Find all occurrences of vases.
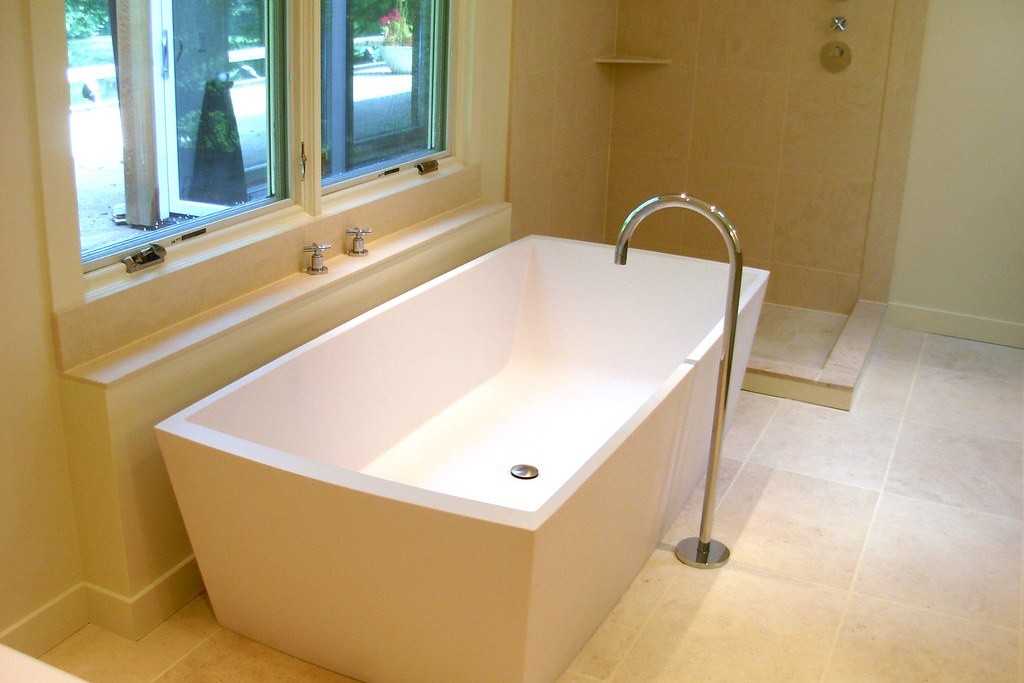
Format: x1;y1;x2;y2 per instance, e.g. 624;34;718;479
379;45;413;74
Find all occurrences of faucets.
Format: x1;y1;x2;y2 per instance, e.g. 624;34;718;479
613;193;742;284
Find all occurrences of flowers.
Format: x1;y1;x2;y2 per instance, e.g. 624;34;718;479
379;10;413;46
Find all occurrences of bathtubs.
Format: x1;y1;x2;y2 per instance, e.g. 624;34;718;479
152;231;772;683
